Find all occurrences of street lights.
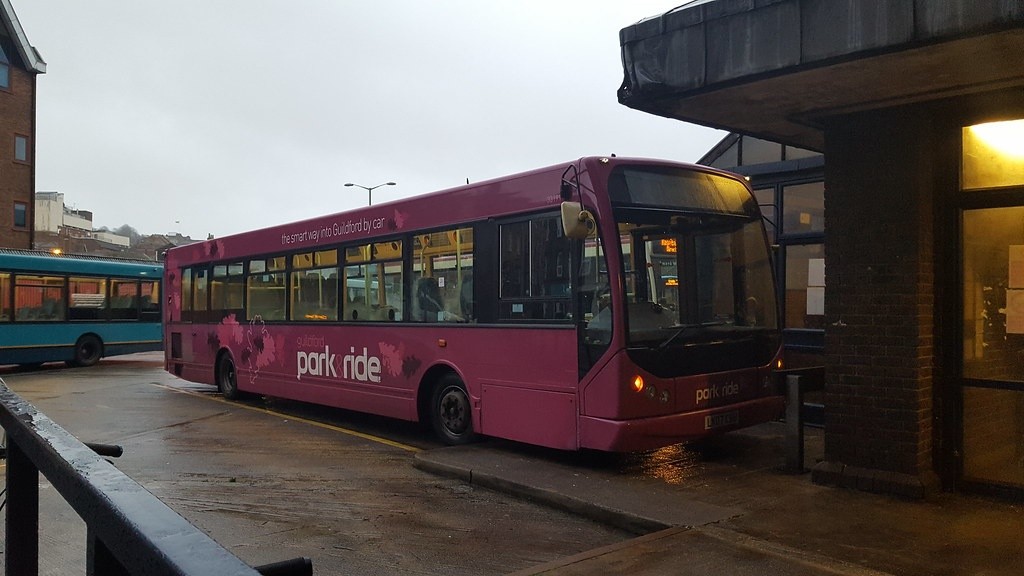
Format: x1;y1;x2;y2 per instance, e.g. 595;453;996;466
344;182;396;205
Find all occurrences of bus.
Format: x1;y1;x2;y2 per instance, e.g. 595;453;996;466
378;230;680;324
0;253;164;369
164;155;786;454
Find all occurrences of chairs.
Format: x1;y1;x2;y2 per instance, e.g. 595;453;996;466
191;274;353;323
17;293;152;321
416;278;446;323
